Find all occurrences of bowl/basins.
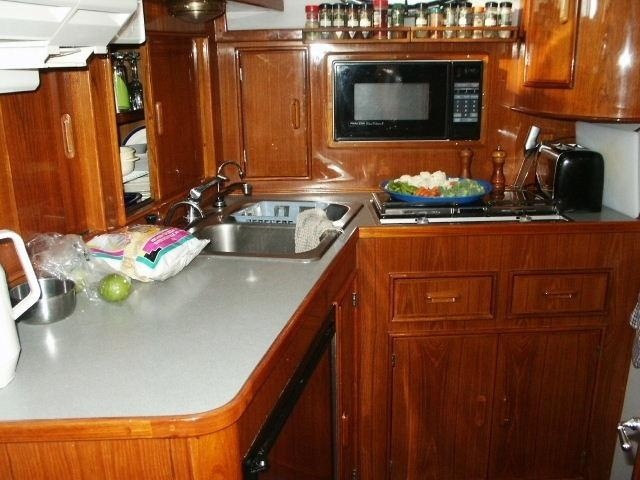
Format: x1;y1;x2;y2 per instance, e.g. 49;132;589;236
8;277;76;325
117;147;140;177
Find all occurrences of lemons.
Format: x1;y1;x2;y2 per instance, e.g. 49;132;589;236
100;274;133;303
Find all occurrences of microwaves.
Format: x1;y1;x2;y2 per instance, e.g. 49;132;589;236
332;59;484;143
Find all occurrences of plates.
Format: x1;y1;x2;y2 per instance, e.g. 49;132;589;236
381;178;493;205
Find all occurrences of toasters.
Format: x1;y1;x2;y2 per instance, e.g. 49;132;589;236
536;143;605;213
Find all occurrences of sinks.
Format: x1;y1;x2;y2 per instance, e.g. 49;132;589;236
229;197;349;226
193;224;326;257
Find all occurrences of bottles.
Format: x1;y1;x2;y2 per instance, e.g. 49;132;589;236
303;0;513;42
110;51;143;110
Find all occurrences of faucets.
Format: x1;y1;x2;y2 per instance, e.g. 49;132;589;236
214;158;244;214
143;199;204;228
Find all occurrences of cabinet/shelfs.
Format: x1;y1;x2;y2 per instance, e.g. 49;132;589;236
0;228;358;480
359;221;639;480
498;0;640;124
1;30;206;242
218;42;313;196
